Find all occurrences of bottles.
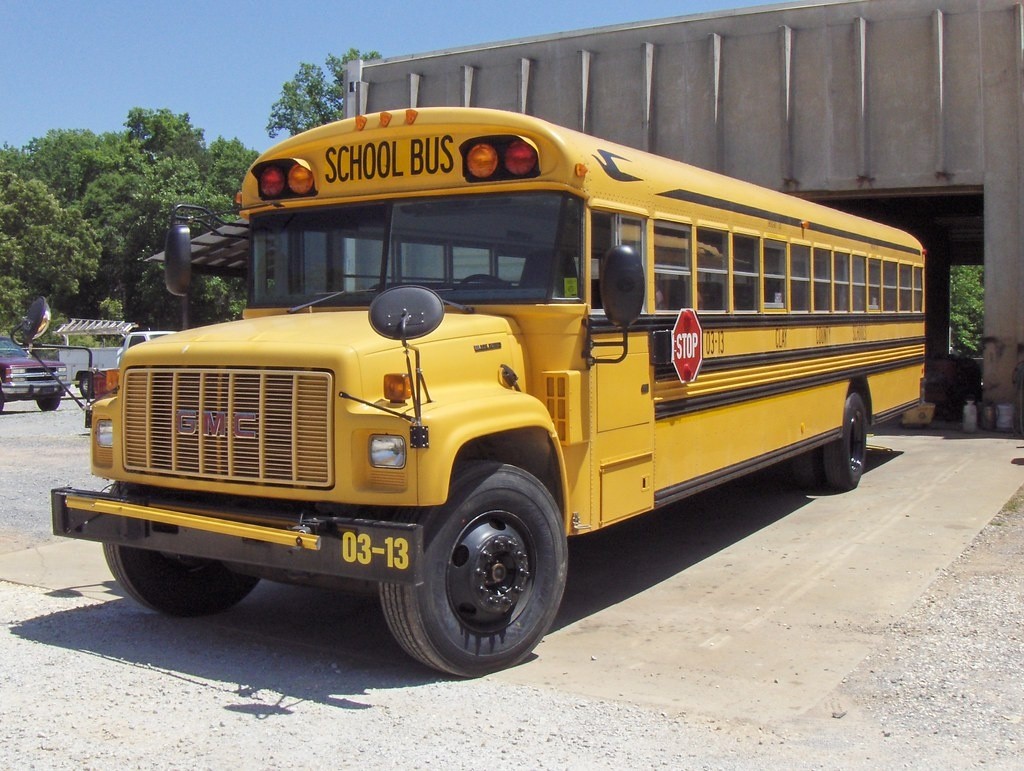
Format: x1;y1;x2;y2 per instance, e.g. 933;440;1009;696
961;395;977;433
982;399;999;431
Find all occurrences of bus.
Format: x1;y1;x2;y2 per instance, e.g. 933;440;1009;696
51;101;930;675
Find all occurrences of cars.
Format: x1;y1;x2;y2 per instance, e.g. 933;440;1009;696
124;330;178;355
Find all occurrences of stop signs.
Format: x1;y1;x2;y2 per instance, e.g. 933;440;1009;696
671;307;702;384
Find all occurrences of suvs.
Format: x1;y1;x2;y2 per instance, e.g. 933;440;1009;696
0;337;73;414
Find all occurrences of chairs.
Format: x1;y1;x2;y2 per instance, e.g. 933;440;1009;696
518;249;785;309
